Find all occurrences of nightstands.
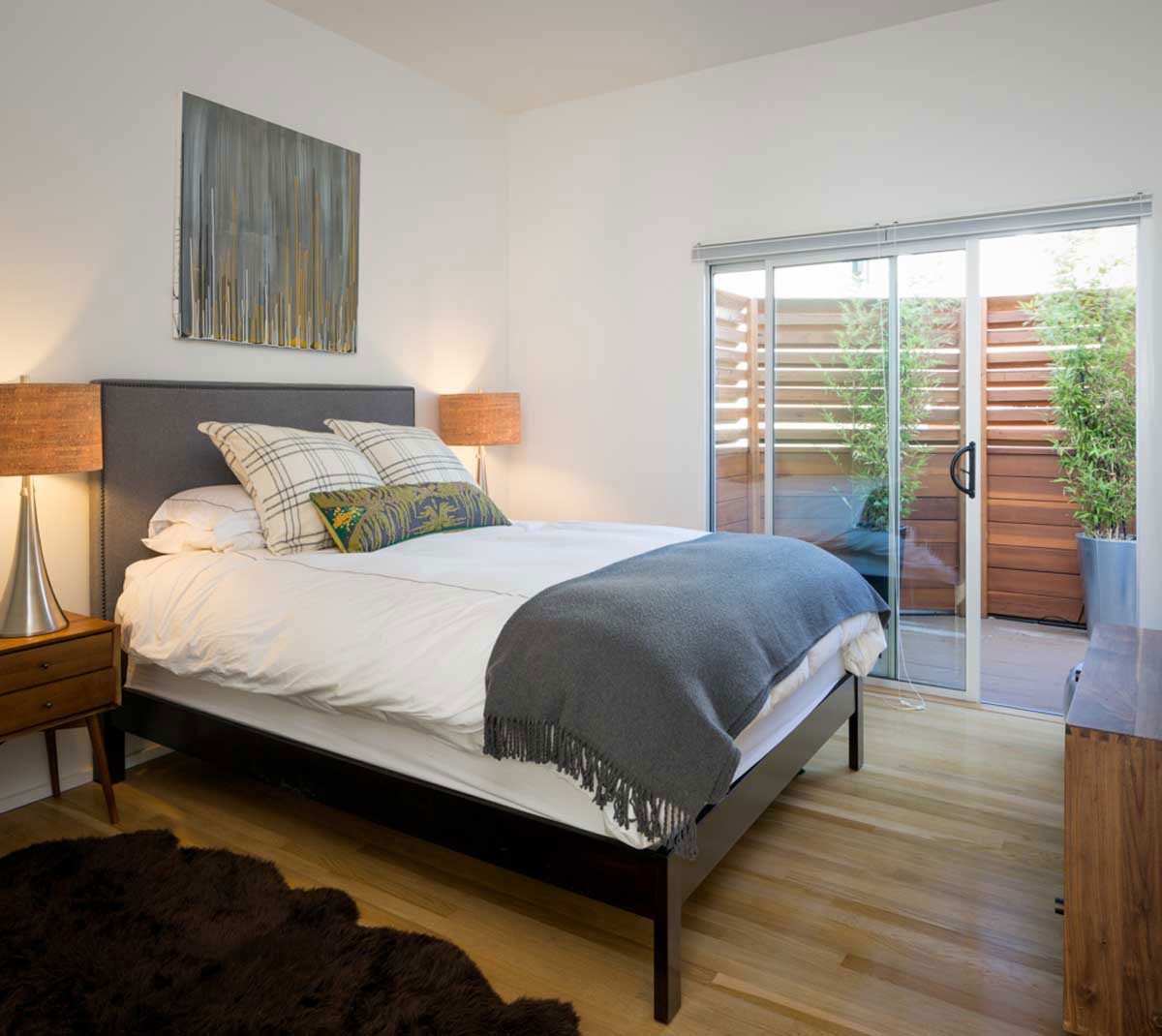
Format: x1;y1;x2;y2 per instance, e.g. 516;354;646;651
0;608;122;825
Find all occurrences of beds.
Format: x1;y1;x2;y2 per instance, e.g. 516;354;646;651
90;381;891;1025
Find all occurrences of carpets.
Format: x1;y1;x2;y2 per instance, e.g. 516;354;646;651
0;829;581;1035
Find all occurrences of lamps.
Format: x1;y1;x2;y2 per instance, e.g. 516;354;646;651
0;374;103;637
439;389;523;498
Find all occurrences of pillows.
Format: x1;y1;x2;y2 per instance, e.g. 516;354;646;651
309;482;513;553
142;485;267;555
198;420;382;554
323;417;482;492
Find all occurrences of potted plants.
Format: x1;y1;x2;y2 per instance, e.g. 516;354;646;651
1012;230;1138;642
809;273;961;630
